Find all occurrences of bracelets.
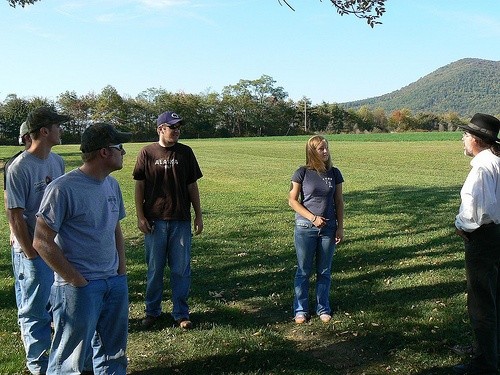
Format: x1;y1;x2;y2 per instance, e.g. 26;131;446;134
312;217;316;222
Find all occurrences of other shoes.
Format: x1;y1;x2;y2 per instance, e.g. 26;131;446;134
292;315;308;323
452;364;472;374
320;314;332;322
81;371;94;375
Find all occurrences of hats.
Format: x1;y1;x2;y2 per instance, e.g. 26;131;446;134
157;111;183;126
453;112;500;142
26;106;75;132
80;123;132;153
20;121;30;138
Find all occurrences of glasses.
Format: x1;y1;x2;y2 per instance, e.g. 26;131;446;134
107;143;122;151
166;123;181;129
463;132;471;138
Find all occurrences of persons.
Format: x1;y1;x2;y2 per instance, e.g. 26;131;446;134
33;123;132;375
4;109;73;375
289;136;345;323
132;112;203;327
455;114;500;375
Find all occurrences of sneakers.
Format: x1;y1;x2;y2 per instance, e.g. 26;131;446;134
177;317;192;328
142;315;158;325
